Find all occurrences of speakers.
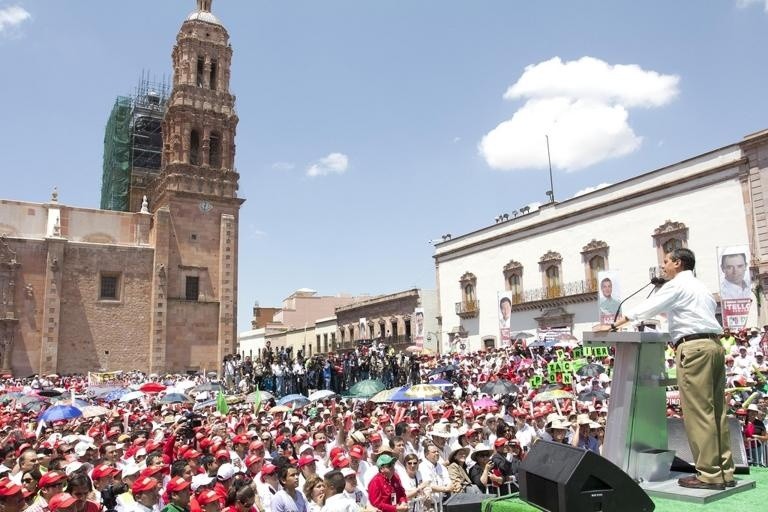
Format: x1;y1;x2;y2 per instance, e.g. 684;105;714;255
520;439;655;510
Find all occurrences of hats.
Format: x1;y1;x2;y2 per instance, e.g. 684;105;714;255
0;376;420;512
725;326;768;417
666;354;674;362
417;344;602;464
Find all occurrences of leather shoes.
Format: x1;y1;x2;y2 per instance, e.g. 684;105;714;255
677;474;737;491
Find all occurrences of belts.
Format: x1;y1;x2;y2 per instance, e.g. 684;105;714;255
674;332;720;350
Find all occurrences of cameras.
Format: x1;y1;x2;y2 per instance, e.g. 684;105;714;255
508;442;517;446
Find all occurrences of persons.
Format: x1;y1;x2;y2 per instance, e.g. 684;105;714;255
1;324;767;511
497;296;512;344
721;253;758;332
591;248;737;491
415;312;425;349
599;277;623;324
360;323;366;339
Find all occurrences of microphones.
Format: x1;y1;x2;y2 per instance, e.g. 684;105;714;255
647;278;666;299
613;277;659;330
